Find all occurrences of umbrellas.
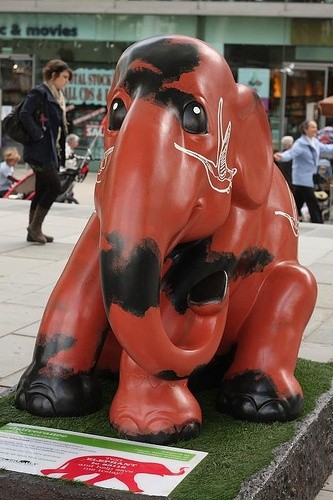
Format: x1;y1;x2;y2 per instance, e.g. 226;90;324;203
318;96;333;116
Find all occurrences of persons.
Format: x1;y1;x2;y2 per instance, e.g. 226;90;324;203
21;59;73;243
59;134;79;196
0;147;21;198
272;120;333;225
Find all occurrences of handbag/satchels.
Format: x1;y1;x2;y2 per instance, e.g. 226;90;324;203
313;174;321;184
1;98;41;146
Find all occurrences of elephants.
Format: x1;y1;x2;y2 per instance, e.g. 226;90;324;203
12;35;318;444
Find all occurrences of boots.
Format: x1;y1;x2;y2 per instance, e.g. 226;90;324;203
27;207;54;242
27;203;49;244
